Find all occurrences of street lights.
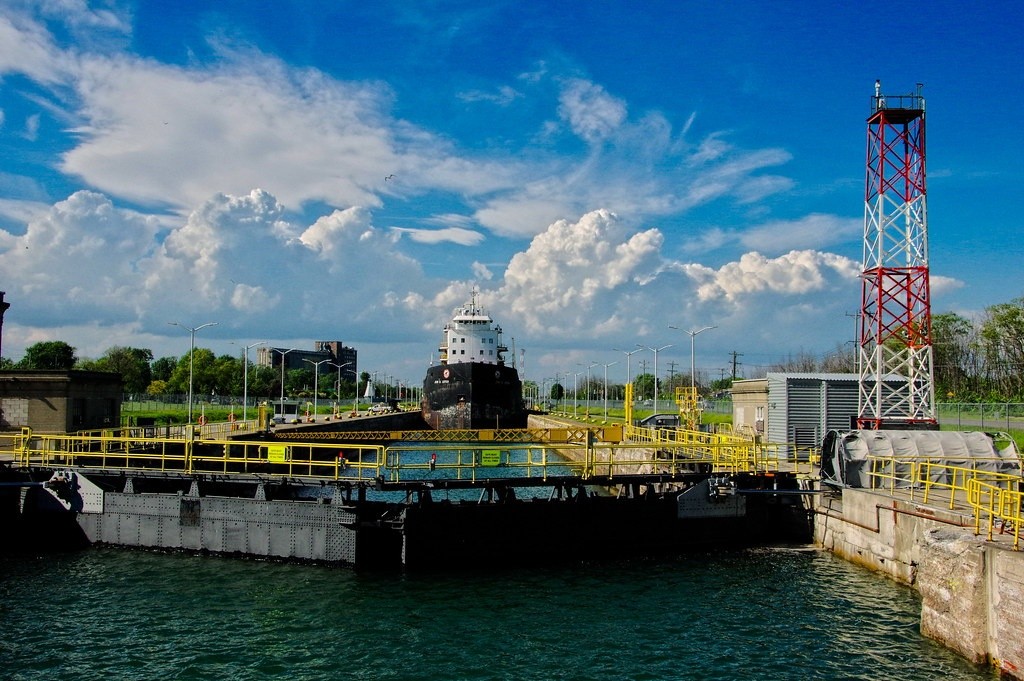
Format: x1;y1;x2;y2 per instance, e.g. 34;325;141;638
347;370;367;413
301;359;332;420
168;323;219;424
269;348;301;417
327;362;352;403
636;344;673;414
576;363;601;411
527;372;583;417
373;371;419;411
592;361;620;421
612;348;644;384
228;342;268;423
669;326;719;401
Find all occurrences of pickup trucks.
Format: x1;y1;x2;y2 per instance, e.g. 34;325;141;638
368;405;392;413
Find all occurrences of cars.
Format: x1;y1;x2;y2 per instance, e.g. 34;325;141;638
626;413;680;441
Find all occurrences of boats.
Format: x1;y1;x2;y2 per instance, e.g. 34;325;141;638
420;287;530;430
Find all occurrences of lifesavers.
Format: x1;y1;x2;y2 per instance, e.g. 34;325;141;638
199;416;208;424
334;409;337;413
305;410;312;417
228;413;238;422
